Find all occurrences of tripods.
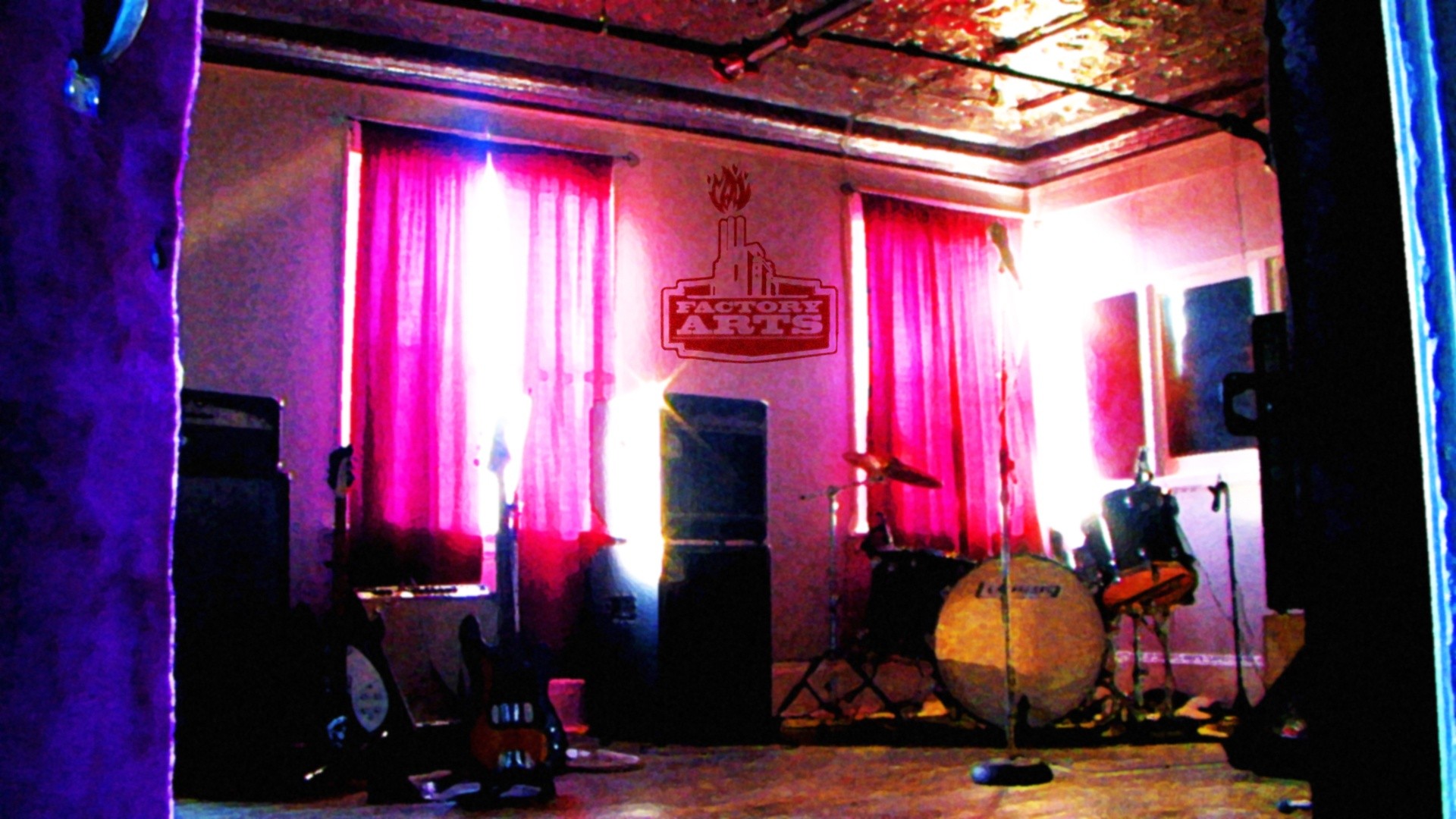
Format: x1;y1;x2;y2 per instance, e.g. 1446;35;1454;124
774;484;906;724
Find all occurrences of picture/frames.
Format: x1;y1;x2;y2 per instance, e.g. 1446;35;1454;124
1049;246;1285;486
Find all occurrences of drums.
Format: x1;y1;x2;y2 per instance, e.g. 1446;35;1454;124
838;482;1199;735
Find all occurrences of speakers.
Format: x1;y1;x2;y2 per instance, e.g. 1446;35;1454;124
179;384;281;473
657;545;772;744
660;393;769;543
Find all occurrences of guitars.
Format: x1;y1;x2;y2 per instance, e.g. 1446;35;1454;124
291;443;438;790
457;499;568;802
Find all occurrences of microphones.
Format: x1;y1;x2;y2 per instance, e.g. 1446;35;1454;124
1131;446;1147;484
1205;478;1227;512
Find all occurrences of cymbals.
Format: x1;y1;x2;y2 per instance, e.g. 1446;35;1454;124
842;450;939;488
